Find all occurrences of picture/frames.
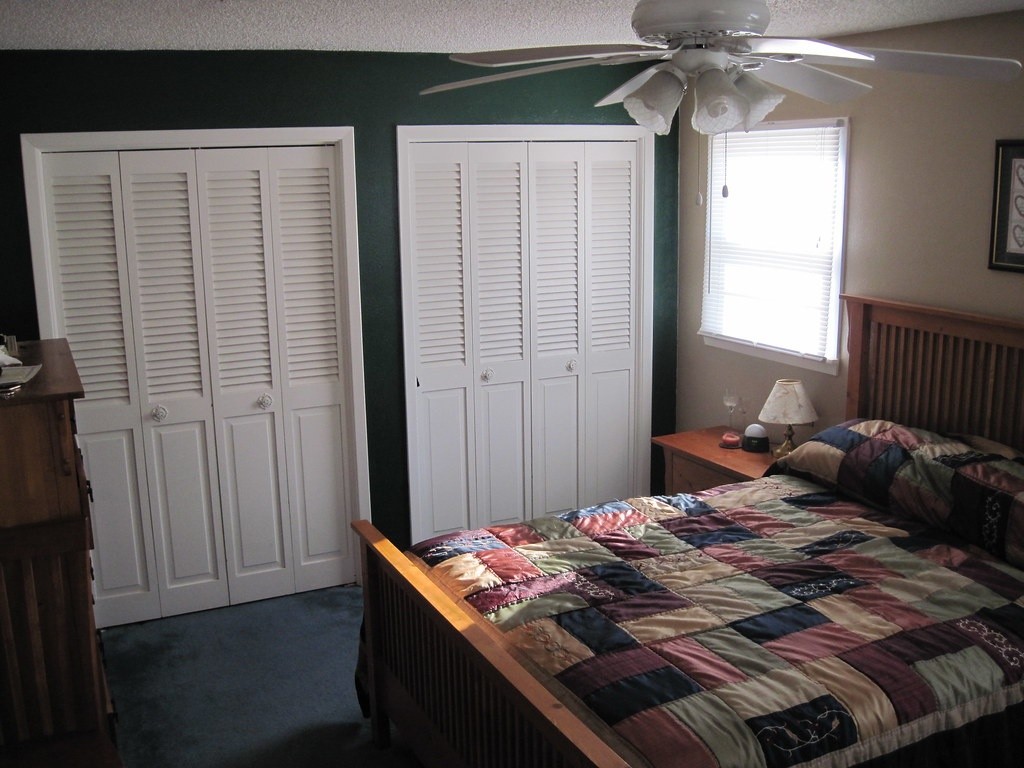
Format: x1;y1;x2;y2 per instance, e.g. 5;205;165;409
989;139;1024;270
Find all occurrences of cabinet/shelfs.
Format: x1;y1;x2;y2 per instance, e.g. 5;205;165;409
1;339;124;768
20;126;372;629
395;124;655;545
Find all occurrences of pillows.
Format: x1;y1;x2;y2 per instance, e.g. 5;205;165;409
763;417;1024;570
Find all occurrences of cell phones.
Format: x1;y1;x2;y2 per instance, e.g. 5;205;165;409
0;385;21;393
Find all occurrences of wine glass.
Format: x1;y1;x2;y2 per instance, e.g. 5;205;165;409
723;384;739;435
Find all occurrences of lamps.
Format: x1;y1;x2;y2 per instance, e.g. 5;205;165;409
757;378;819;458
623;48;786;206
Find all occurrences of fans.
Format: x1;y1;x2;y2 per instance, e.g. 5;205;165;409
419;0;1022;106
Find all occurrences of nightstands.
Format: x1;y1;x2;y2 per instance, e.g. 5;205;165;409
651;425;782;496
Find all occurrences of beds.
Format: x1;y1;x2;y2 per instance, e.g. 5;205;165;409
353;295;1024;768
